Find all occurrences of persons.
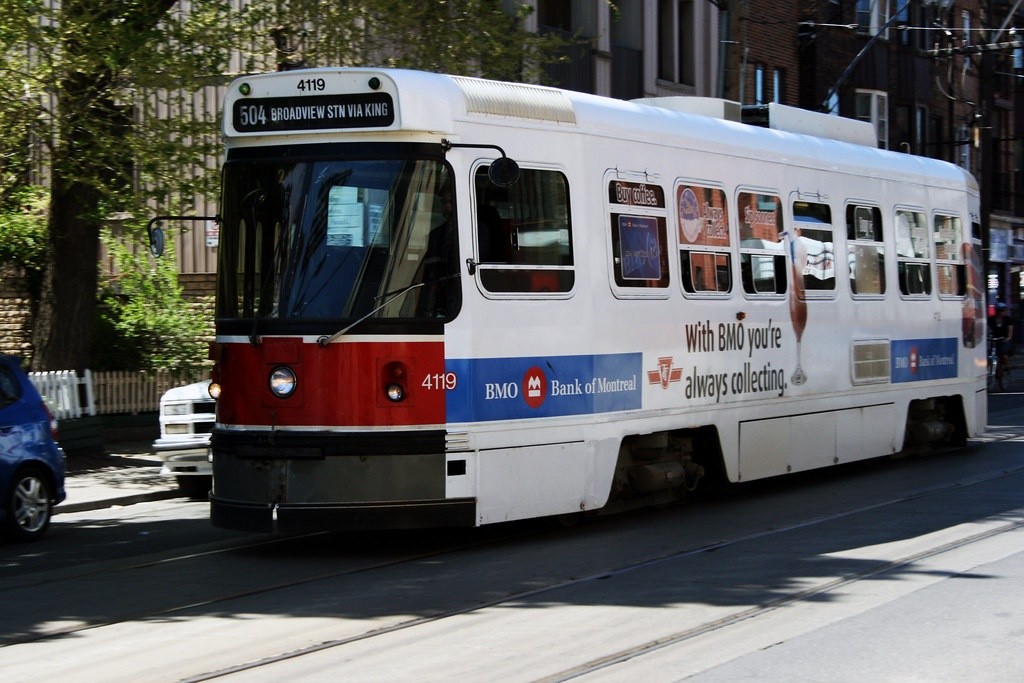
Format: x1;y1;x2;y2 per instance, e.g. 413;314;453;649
425;172;513;321
987;302;1014;370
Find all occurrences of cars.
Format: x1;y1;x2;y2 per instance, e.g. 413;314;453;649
152;380;212;503
0;352;66;547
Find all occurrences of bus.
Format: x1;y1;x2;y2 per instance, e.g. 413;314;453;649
147;67;988;537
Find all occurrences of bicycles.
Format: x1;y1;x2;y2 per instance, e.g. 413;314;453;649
985;335;1012;394
